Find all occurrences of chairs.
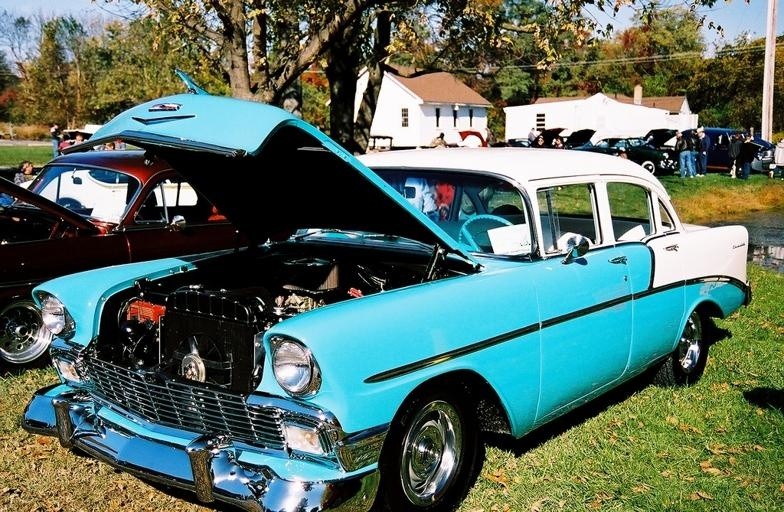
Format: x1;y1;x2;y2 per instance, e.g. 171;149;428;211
490;204;524;227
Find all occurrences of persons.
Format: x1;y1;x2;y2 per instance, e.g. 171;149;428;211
527;128;783;180
431;182;456;222
13;160;37;187
49;120;125;158
430;132;449;148
403;177;441;224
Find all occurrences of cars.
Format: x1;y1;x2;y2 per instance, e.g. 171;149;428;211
15;161;197;225
0;149;248;368
59;123;107;152
22;67;751;511
